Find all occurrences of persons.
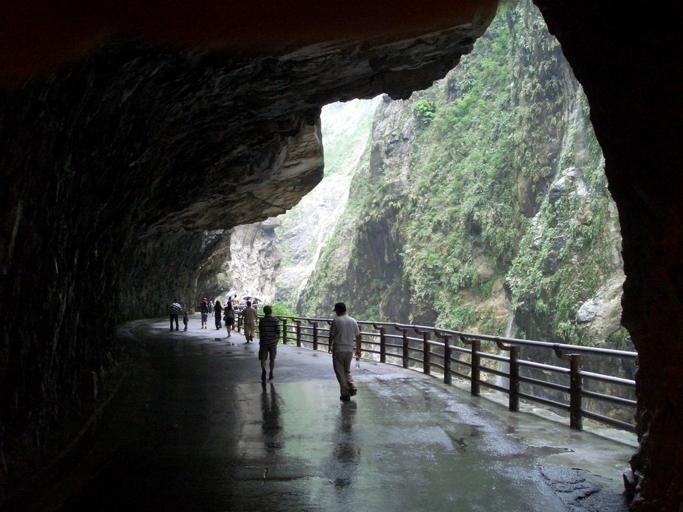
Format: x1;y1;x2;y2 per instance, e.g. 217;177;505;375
328;303;362;402
168;294;258;343
259;306;280;381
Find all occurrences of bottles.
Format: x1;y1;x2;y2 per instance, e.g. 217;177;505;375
356;354;359;369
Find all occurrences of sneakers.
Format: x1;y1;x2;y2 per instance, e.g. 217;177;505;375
351;388;357;396
247;335;253;343
340;396;350;401
262;370;273;380
